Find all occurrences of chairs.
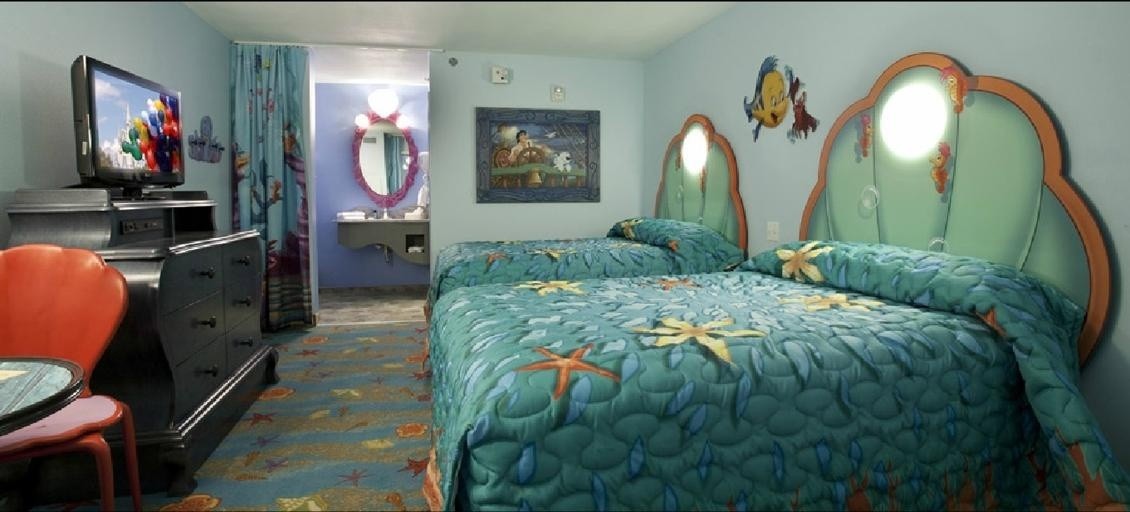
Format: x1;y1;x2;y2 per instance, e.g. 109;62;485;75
0;243;143;512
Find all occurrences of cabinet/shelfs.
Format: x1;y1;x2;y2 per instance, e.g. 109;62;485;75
5;188;281;496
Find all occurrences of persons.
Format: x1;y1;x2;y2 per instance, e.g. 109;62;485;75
508;129;536;161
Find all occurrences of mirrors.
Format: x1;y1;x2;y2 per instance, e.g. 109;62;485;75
351;111;419;208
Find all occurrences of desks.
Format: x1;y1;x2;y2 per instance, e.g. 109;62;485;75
0;353;86;436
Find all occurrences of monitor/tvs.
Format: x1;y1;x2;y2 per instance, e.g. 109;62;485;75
69;55;185;201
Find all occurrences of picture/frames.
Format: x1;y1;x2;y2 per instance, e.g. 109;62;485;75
474;105;601;204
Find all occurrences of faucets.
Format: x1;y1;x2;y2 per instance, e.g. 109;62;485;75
383;207;389;218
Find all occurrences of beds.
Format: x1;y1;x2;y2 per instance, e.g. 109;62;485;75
423;52;1111;511
435;112;748;297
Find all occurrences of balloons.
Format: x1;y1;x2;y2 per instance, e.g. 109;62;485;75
120;93;182;174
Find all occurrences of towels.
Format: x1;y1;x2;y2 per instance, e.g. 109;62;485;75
336;210;365;221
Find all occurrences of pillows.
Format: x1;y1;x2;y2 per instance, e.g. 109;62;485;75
607;217;746;274
733;238;1130;511
